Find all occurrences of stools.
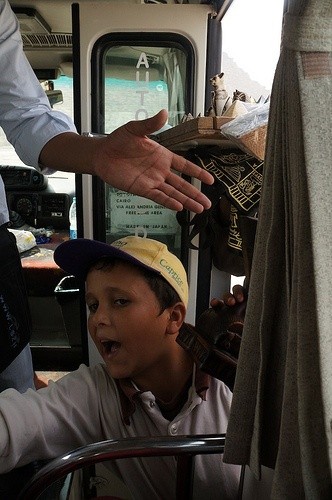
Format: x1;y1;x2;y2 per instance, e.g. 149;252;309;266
19;228;78;349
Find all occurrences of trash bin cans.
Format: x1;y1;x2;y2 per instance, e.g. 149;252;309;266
54;275;82;347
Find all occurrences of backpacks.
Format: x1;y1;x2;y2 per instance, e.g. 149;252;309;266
176;145;264;276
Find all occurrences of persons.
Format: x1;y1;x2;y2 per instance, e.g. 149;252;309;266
0;236;243;500
0;0;214;499
210;284;243;308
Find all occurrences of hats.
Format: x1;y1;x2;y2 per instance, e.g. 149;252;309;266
53;236;188;312
